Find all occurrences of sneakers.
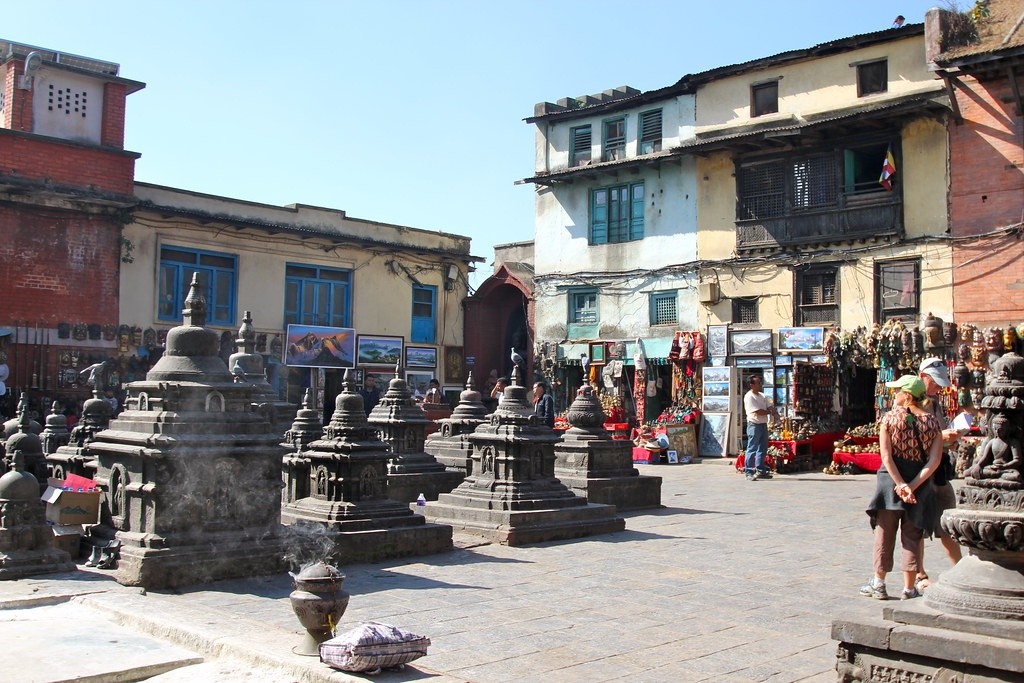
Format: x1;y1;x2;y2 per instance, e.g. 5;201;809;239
899;590;921;601
859;578;888;599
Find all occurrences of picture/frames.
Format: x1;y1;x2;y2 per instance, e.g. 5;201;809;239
405;370;433;396
443;345;464;383
591;342;608;366
356;335;404;369
665;324;824;462
363;372;397;397
280;324;356;370
405;345;437;367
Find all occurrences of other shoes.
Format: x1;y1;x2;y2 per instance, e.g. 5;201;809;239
920;575;929;589
755;472;774;479
746;473;757;481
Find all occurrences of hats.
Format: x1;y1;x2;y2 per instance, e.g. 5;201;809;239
885;375;926;402
920;357;952;387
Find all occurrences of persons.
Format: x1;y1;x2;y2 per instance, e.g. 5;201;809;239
64;406;78;429
360;373;383;417
911;356;963;596
422;377;449;404
491;377;511;405
862;374;944;603
742;375;780;481
532;382;555;428
102;386;118;412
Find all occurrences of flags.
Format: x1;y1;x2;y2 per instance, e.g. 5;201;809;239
879;142;896;191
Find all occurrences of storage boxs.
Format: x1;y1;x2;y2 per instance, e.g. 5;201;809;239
40;477;104;526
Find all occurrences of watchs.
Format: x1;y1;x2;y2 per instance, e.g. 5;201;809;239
768;409;773;413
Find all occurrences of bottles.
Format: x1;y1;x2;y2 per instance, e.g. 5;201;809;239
416;493;426;515
56;486;94;492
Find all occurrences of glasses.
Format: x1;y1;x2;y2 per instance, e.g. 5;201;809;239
923;361;947;372
